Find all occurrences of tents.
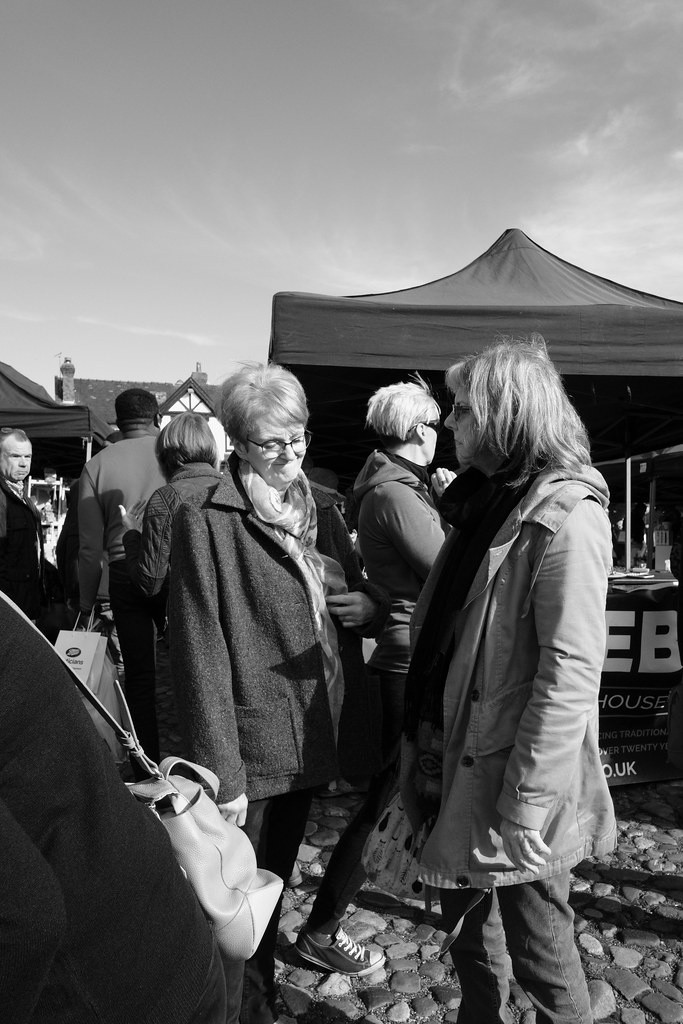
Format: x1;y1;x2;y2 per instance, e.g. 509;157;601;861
0;361;118;462
266;228;683;573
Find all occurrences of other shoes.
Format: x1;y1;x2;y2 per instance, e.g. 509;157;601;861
288;860;303;887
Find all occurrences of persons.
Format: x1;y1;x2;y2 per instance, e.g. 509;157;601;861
0;342;617;1024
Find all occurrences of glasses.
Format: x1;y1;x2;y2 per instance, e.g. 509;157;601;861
452;404;473;421
409;421;441;435
247;429;312;458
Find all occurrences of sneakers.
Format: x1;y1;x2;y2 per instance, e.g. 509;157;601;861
294;922;387;976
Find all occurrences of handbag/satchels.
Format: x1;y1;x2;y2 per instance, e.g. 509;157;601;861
123;755;284;961
361;792;441;902
54;604;108;694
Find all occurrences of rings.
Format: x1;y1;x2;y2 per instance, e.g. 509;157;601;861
522;848;533;855
135;516;138;519
